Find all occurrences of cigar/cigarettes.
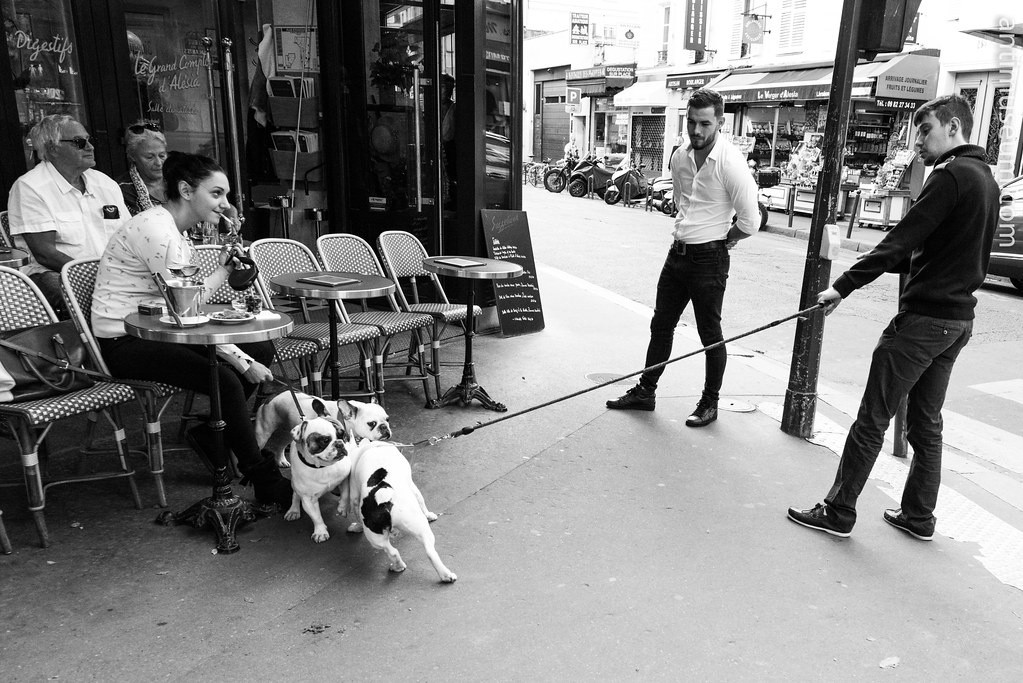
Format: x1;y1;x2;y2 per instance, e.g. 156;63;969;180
219;212;236;226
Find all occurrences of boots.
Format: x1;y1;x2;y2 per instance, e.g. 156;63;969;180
239;449;293;514
184;422;234;482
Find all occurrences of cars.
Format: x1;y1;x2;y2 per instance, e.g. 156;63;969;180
987;175;1023;292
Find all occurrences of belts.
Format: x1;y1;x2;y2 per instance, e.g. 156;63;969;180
674;240;726;255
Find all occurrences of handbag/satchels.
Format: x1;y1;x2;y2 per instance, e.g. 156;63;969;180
0;319;95;404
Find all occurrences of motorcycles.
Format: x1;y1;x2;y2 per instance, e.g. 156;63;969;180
544;138;782;230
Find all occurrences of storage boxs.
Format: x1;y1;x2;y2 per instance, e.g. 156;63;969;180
137;303;166;316
757;166;781;186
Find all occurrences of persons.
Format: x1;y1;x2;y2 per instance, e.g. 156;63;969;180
786;93;999;538
7;114;132;323
111;121;171;217
606;87;762;428
93;149;294;512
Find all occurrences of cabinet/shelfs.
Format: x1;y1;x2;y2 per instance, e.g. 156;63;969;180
747;131;888;164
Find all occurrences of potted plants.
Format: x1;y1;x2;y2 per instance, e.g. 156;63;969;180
365;29;423;105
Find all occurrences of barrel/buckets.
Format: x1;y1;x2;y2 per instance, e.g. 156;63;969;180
164;279;205;317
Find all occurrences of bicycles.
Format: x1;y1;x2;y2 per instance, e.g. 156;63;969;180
522;156;566;188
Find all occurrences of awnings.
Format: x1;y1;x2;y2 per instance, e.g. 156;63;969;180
614;80;673;107
699;49;940;101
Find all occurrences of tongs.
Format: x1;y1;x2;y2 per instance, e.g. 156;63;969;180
151;272;183;326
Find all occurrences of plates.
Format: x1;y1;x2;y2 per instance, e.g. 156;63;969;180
206;312;254;324
159;316;210;328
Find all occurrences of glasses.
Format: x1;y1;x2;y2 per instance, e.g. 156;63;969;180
128;123;163;134
60;138;97;149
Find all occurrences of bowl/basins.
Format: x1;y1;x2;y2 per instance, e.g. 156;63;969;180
231;299;248;311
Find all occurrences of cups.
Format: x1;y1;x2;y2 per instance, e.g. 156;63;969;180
244;294;262;315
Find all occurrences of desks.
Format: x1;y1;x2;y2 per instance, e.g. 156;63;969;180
0;247;31;271
122;304;293;553
423;254;524;412
269;271;395;402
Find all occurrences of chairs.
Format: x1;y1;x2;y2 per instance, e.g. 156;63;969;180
376;228;481;393
0;201;138;254
315;234;437;410
249;238;385;422
0;266;168;549
60;258;189;508
192;241;321;422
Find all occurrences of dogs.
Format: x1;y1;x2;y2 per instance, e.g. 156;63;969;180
284;400;357;542
353;439;457;583
255;390;393;468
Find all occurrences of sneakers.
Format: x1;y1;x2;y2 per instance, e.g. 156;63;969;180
606;384;655;411
787;503;851;537
686;395;718;427
883;508;933;541
